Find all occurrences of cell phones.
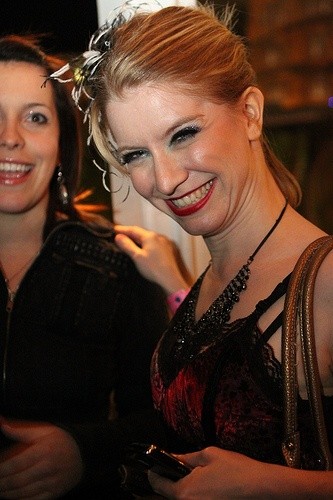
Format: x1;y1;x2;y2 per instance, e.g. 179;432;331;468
122;442;193;482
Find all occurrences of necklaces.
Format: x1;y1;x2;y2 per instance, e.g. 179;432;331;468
1;246;42;284
172;197;288;360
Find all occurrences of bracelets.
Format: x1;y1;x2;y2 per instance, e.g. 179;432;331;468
167;288;192;312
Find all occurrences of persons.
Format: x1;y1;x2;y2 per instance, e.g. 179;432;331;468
84;5;333;500
0;34;170;500
